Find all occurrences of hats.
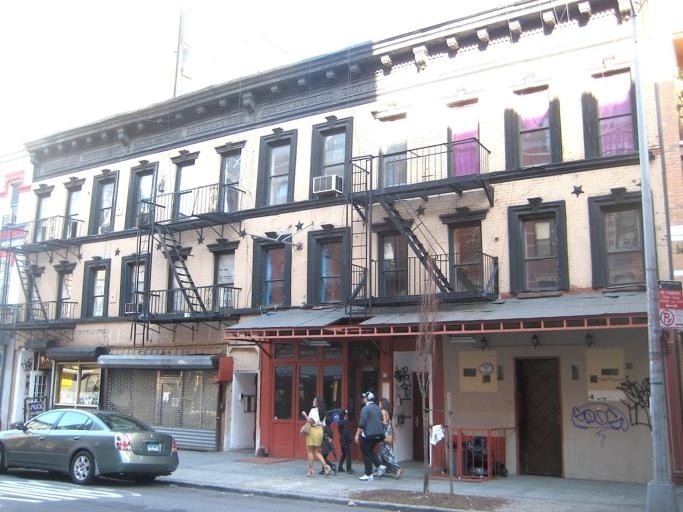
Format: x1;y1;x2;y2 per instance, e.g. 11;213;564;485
362;392;374;400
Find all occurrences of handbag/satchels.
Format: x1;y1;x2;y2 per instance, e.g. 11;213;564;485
383;434;392;444
300;423;311;435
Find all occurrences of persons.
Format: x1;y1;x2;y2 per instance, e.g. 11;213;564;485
335;407;355;475
299;395;332;479
372;396;404;480
353;390;386;482
319;415;339;475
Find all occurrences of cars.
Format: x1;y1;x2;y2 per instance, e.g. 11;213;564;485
0;407;181;485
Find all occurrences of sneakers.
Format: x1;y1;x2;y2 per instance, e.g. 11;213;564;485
395;469;402;479
358;474;372;481
376;466;386;478
306;464;354;477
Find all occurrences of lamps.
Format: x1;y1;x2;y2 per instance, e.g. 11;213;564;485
451;334;476;342
481;337;487;352
532;334;541;349
586;333;593;347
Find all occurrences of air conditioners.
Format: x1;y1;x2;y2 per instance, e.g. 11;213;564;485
313;176;343;194
125;302;143;314
